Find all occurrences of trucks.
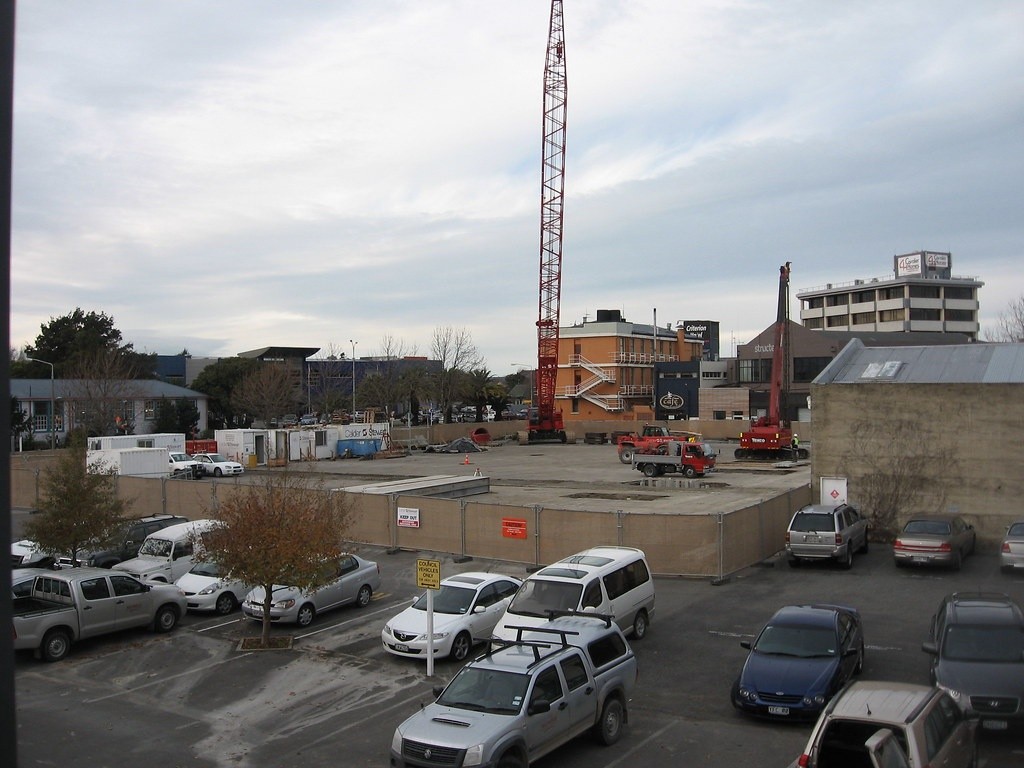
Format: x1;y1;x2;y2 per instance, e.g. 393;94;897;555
630;441;721;478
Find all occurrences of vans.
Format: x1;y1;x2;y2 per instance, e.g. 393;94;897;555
490;545;657;651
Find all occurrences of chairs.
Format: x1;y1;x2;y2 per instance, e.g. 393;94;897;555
484;672;516;703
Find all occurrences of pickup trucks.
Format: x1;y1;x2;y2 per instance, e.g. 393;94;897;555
11;565;188;662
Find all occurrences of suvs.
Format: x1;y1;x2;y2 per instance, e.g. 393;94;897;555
794;679;977;768
388;609;639;768
11;513;234;585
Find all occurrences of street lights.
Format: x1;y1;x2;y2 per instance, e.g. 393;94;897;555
510;363;533;409
349;339;358;422
24;357;55;449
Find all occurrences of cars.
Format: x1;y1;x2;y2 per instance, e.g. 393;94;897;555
920;590;1024;733
381;570;525;661
264;405;529;429
171;549;256;613
736;603;862;722
997;521;1024;573
169;452;244;476
785;503;870;570
894;515;978;572
242;552;382;628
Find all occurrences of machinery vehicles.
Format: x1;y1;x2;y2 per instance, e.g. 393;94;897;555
735;261;812;461
515;0;576;447
616;424;678;465
